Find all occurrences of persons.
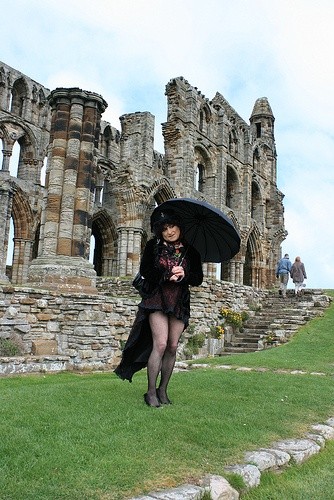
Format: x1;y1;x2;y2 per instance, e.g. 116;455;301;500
113;217;204;408
290;257;307;296
276;254;292;298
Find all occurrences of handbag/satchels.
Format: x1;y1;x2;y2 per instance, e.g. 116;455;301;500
132;270;159;299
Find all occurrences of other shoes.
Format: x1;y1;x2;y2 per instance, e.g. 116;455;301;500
278;290;282;295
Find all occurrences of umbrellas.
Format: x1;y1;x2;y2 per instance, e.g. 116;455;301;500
150;198;241;282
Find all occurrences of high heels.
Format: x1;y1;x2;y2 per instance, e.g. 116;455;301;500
155;387;172;405
144;393;162;408
